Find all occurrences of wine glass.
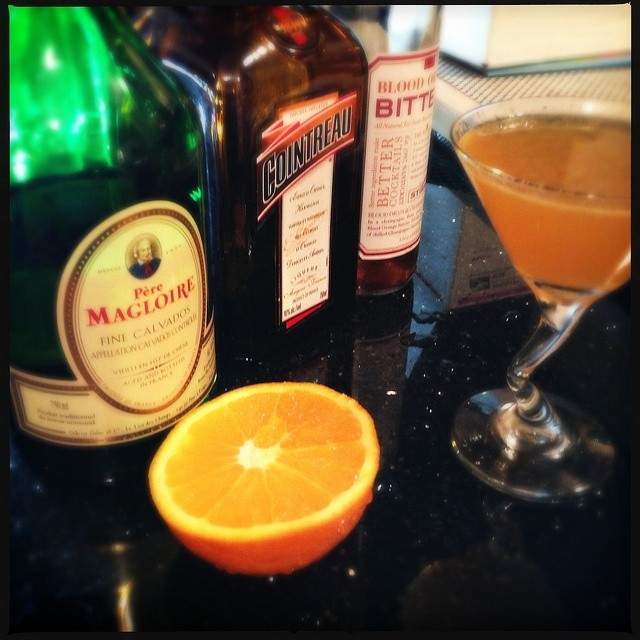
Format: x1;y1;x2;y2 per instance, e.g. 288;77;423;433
449;96;631;503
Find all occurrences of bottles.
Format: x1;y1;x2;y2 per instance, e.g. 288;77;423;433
7;3;223;498
318;2;445;298
131;5;368;346
